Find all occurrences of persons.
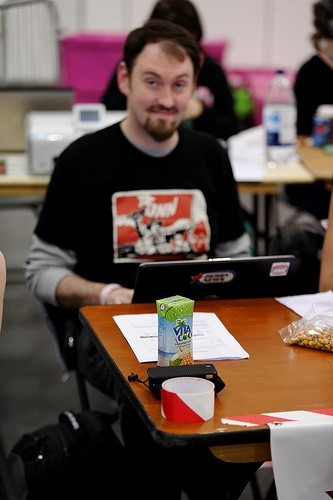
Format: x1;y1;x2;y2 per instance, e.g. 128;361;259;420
24;21;264;500
100;1;238;140
283;1;332;292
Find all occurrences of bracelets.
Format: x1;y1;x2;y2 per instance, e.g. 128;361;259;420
98;283;120;306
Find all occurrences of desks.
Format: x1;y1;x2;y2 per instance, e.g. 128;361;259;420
0;136;333;447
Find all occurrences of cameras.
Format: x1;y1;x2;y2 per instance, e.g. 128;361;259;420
146;363;225;399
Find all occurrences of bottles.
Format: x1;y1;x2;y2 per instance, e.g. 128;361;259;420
262;70;296;170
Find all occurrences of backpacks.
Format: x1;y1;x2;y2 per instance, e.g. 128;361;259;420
0;408;132;500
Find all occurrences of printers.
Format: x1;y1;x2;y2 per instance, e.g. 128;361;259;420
25;104;130;176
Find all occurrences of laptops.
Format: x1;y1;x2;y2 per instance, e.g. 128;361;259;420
132;255;296;304
